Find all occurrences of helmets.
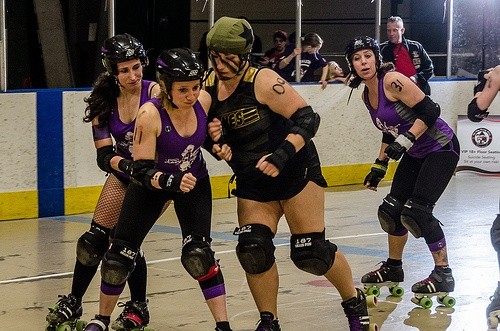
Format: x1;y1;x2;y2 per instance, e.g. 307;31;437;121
206;17;254;55
100;34;149;76
157;48;204;83
343;36;381;72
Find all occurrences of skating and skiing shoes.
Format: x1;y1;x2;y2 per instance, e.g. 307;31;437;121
111;299;151;331
45;293;86;331
82;314;111;331
486;296;500;329
411;265;456;308
254;311;282;331
341;287;379;331
360;260;405;298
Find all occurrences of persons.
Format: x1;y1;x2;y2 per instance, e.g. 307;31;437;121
84;46;237;331
258;32;349;91
44;35;164;331
203;15;370;331
468;60;500;124
380;14;436;99
345;35;460;294
